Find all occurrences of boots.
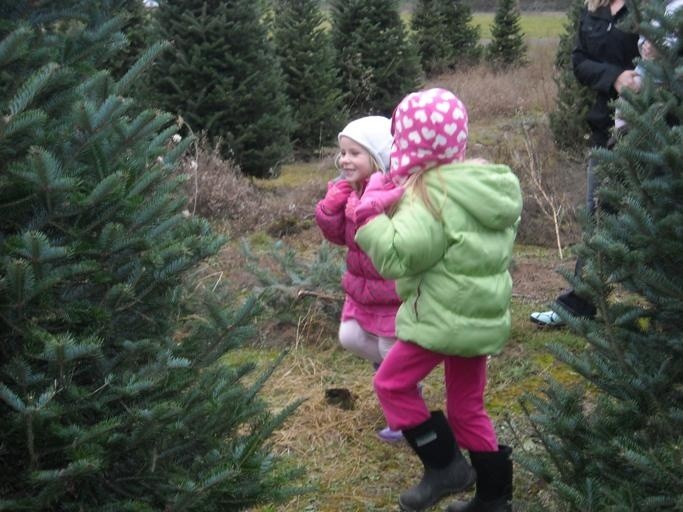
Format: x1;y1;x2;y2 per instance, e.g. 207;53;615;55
399;409;477;512
446;445;512;512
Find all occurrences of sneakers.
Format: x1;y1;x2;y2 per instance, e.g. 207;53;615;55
379;426;402;440
530;311;561;327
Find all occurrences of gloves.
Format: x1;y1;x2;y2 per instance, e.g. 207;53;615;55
322;172;404;223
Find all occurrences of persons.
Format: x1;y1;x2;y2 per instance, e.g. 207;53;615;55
605;2;683;141
312;116;429;442
529;1;663;329
352;86;523;511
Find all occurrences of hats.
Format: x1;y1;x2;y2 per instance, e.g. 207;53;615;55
390;89;468;169
337;115;389;176
